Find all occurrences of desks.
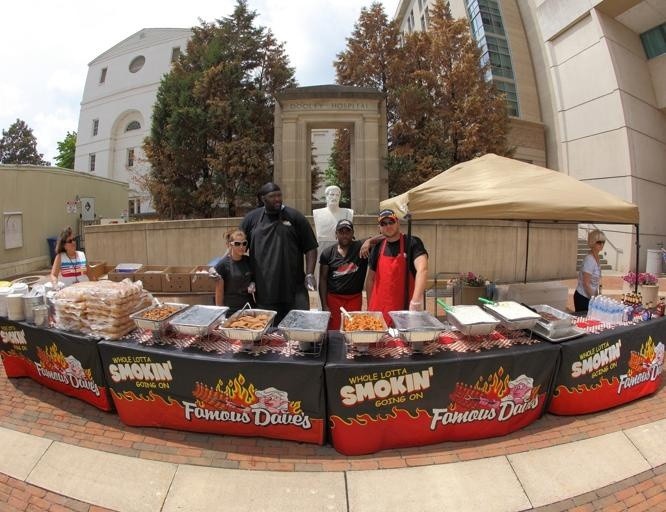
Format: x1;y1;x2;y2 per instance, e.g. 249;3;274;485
150;291;216;306
544;307;666;417
322;329;563;458
96;328;330;447
0;316;137;413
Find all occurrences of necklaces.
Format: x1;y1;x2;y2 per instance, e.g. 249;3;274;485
66;252;77;281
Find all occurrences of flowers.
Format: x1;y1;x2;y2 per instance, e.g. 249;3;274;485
621;269;659;286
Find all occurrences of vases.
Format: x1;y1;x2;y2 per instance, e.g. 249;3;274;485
640;284;659;309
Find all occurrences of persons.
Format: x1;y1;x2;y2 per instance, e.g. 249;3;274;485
364;209;429;314
208;182;319;326
50;226;97;297
318;219;387;330
312;185;354;267
574;229;607;312
213;228;257;318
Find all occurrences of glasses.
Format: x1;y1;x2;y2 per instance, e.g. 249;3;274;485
595;241;605;244
379;220;396;227
229;240;248;248
65;237;76;243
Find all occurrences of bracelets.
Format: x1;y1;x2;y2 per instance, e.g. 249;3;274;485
410;300;423;306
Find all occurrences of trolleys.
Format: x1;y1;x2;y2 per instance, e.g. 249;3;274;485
423;271;463;326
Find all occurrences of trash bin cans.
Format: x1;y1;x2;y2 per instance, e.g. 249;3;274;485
46;237;57;265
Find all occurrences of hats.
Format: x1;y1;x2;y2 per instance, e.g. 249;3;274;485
336;219;354;232
377;209;398;224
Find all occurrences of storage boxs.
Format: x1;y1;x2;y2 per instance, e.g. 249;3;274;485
161;265;196;292
86;260;109;282
190;264;216;292
135;264;167;292
108;265;143;283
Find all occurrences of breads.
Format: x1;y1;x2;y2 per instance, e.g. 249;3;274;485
51;278;153;343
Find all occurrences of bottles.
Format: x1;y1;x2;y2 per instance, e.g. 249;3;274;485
587;295;628;325
621;291;665;321
